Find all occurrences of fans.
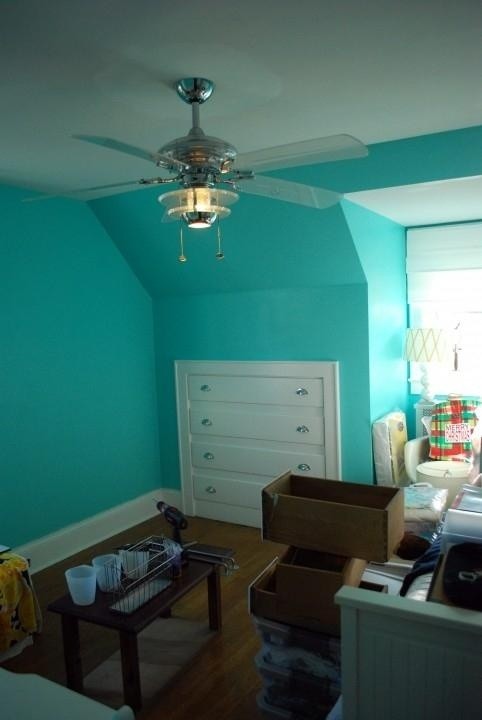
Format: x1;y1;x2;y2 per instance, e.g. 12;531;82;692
18;75;367;263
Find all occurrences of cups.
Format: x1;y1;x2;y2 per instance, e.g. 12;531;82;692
64;544;150;605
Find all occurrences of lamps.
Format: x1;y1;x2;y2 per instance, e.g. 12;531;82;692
157;186;240;232
404;328;448;404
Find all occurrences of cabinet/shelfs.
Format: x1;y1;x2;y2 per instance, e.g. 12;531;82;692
175;359;339;527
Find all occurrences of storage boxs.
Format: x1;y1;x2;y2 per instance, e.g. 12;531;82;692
247;470;405;719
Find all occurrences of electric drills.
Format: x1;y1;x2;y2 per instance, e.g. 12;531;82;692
151;496;188;567
